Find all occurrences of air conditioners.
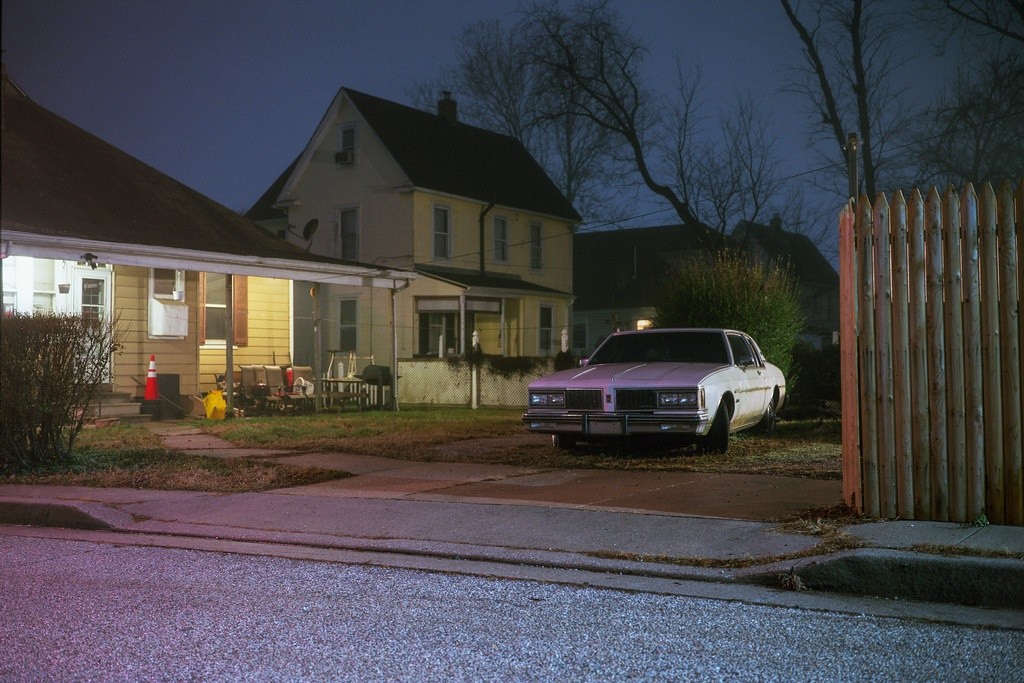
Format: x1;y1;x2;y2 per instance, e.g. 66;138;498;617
335;150;352;164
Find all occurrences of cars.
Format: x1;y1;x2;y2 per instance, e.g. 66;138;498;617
521;328;786;454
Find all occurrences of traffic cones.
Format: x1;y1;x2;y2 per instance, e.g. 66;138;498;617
138;356;162;404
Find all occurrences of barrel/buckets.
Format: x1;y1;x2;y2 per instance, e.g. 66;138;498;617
172;284;184;301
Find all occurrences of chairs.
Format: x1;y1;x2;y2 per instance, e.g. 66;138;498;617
239;364;327;416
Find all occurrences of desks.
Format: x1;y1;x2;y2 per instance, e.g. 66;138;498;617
313;378;364;412
